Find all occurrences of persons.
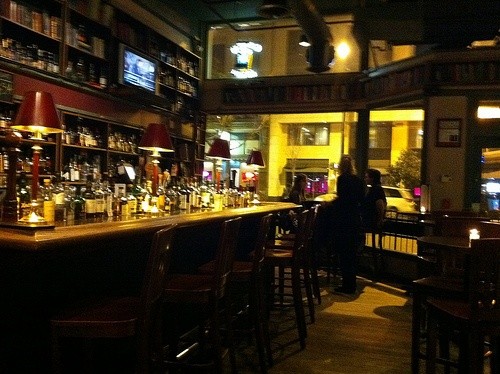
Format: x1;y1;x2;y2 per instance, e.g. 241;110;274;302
333;153;365;293
363;168;387;227
285;173;309;228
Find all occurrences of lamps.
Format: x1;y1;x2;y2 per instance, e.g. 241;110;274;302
10;89;64;224
246;150;265;204
207;139;232;193
140;120;177;214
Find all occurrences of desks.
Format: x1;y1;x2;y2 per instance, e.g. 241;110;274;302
417;235;497;274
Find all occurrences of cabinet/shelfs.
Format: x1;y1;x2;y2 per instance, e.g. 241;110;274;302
0;0;204;187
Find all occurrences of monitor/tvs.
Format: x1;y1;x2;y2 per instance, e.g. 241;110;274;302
118;43;160;97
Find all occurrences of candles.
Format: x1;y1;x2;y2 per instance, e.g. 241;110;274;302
469;229;480;249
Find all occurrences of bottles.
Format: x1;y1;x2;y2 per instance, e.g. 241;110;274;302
1;0;255;228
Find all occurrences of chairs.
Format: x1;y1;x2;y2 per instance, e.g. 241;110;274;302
410;237;500;374
49;200;386;374
